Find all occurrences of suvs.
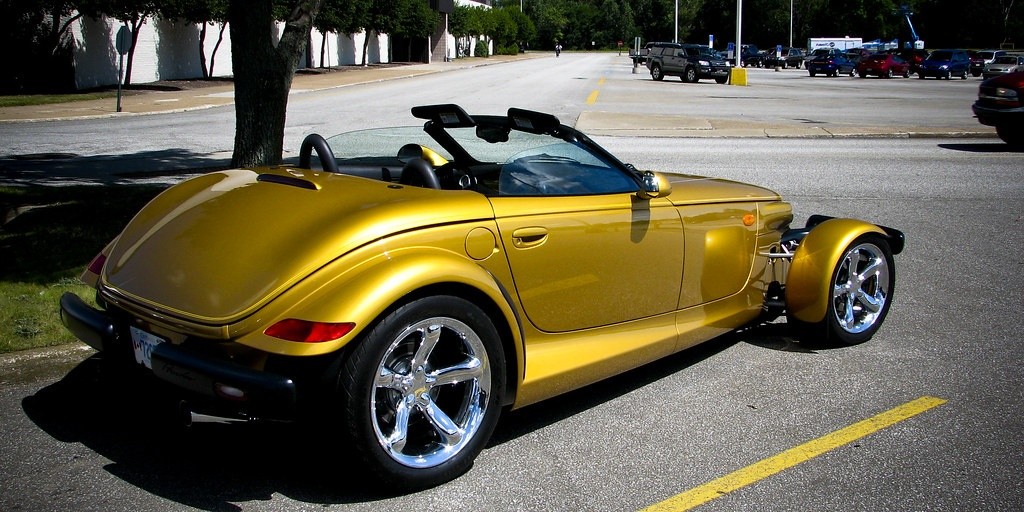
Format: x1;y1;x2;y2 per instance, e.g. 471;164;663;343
766;48;804;69
880;47;930;77
804;48;842;70
971;72;1024;153
981;49;1008;70
646;44;731;84
964;50;985;77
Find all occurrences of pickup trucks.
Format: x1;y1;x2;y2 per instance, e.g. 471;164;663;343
628;42;671;65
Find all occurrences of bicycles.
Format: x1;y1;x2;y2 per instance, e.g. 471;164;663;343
555;48;561;56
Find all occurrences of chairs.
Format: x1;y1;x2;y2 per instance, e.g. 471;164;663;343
299;133;444;190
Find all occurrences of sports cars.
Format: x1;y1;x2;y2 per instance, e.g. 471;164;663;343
57;105;903;488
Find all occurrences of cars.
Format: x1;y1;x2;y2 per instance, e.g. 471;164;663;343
519;45;525;53
919;50;971;80
982;55;1024;79
719;44;775;68
808;54;858;77
858;54;911;79
841;48;875;59
